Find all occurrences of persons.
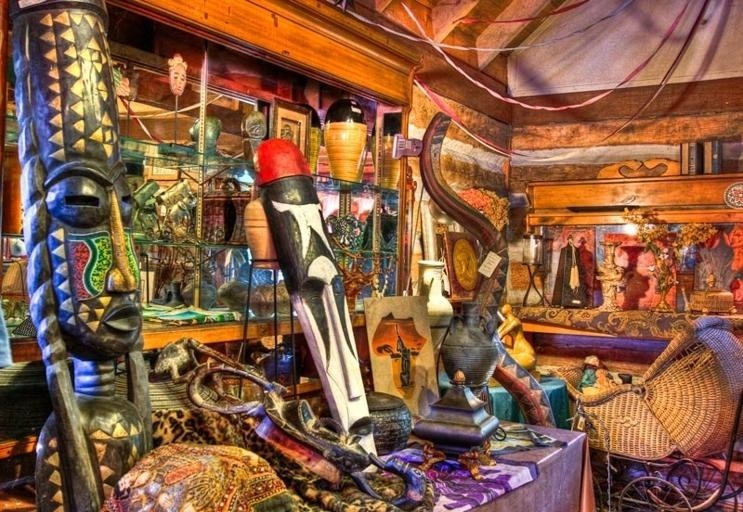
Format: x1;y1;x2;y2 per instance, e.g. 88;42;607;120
168;55;188;96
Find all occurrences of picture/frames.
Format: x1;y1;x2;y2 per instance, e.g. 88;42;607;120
270;93;312;169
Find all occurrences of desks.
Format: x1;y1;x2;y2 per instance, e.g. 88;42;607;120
311;412;588;512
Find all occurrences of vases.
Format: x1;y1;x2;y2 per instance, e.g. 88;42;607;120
322;99;368;184
243;196;281;270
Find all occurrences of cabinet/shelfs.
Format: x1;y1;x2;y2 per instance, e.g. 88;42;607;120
0;0;426;363
